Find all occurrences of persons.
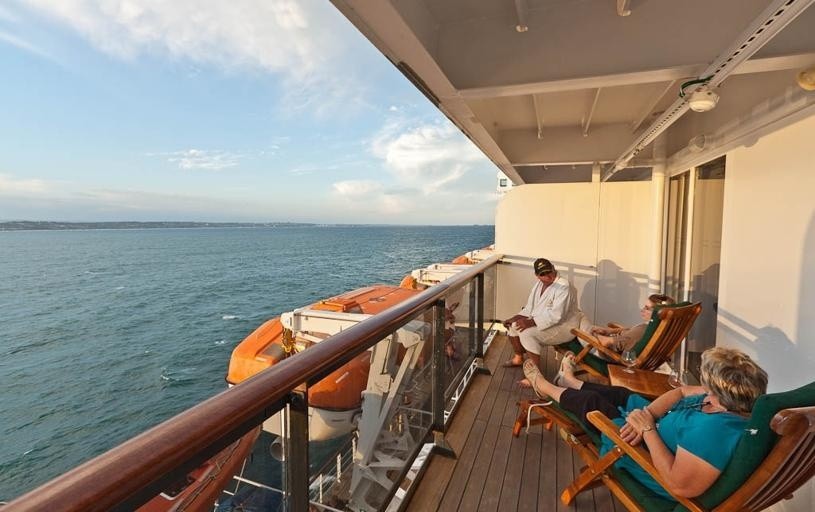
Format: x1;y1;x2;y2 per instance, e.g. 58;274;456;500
522;345;770;501
578;294;675;362
501;258;584;387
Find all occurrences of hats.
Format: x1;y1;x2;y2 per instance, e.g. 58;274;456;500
532;255;554;276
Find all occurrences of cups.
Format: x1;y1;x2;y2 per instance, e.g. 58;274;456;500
667;366;689;388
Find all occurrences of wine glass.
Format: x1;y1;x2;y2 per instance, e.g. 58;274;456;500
620;348;637;374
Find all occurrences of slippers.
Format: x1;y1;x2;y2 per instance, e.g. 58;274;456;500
502;358;523;367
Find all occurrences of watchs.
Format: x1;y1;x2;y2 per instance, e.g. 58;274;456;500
641;424;658;435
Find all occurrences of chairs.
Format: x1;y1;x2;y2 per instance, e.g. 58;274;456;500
512;395;814;511
554;302;702;388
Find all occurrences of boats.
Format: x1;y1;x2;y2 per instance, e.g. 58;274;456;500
222;277;455;443
400;240;497;331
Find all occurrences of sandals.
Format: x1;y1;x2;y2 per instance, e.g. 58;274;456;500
523;358;550;402
553;350;577;387
